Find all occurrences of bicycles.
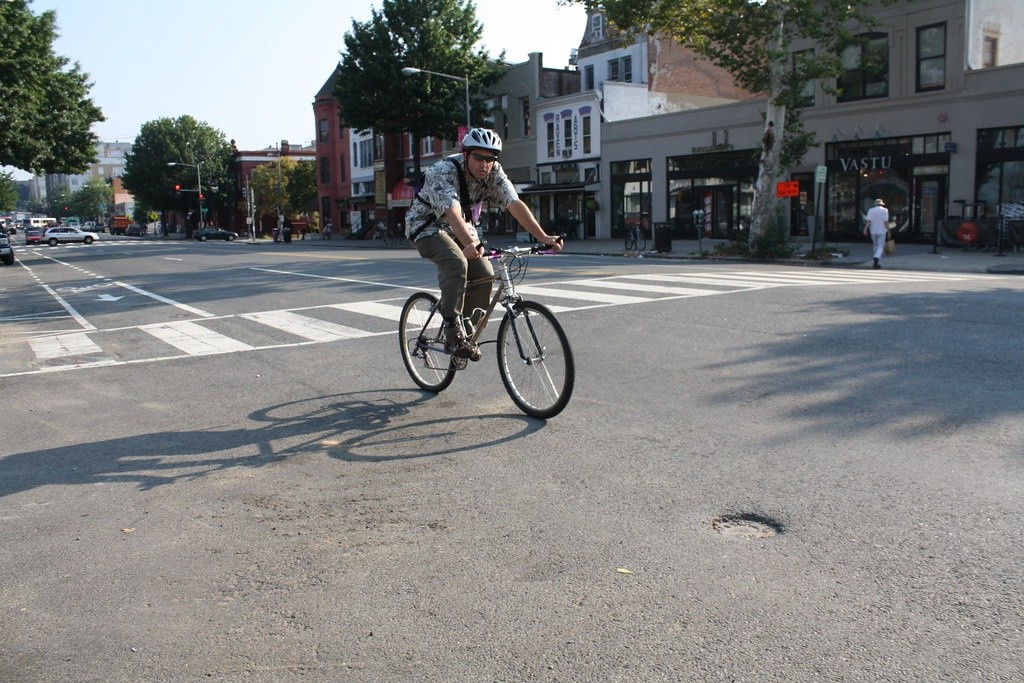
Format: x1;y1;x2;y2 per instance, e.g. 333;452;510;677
398;233;576;421
624;222;648;252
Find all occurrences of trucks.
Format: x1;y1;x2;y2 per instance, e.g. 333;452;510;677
110;216;134;235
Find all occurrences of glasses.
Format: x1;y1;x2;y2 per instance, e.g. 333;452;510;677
468;152;495;163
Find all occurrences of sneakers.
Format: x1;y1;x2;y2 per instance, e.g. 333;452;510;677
467;332;481;361
444;332;480;360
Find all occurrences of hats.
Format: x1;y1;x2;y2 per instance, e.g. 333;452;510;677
872;198;884;205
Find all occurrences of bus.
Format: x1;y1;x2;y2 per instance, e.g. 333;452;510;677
21;217;57;229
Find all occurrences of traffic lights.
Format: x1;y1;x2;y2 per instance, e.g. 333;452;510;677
252;205;257;213
174;183;182;199
65;207;68;214
199;194;206;206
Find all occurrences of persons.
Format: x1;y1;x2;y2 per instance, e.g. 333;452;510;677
405;128;564;362
387;223;405;246
863;199;891;269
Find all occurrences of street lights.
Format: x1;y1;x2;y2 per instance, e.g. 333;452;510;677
167;161;203;229
403;65;473;136
266;151;287;241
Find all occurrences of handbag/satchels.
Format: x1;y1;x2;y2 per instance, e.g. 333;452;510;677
885;240;895;255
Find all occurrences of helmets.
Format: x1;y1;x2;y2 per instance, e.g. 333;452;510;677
461;127;502;155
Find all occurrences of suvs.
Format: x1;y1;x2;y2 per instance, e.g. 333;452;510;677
40;227;100;245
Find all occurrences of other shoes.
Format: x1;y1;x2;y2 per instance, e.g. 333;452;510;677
873;257;881;269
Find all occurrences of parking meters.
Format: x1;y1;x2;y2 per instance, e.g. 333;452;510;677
692;209;706;257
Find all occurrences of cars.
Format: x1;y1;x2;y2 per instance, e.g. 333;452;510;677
125;224;145;236
26;230;42;245
0;234;15;264
196;224;239;242
0;217;22;235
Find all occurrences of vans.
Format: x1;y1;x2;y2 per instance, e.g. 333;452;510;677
60;217;106;233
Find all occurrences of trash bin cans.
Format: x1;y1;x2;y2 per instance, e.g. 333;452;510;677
272;228;279;241
284;228;291;242
654;222;672;252
